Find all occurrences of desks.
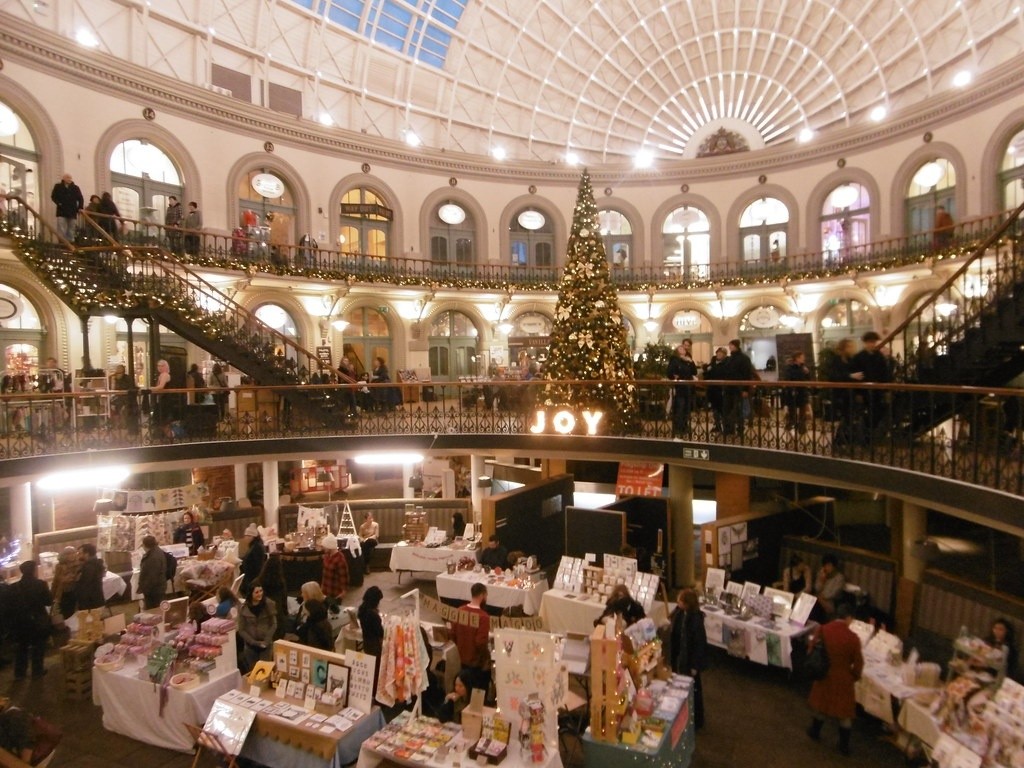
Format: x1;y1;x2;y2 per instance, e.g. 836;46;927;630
1;532;1024;768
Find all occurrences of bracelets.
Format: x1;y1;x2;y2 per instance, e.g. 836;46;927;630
176;223;179;225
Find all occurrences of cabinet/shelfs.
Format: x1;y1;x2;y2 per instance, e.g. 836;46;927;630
944;625;1009;703
71;369;110;443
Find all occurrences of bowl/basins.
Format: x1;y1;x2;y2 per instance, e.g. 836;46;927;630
169;673;200;690
94;654;122;671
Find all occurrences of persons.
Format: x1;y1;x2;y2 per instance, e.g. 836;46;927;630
782;553;845;613
966;617;1015;677
488;355;544;411
110;359;230;441
666;338;698;439
87;192;124;241
312;357;389;412
0;493;707;768
184;201;203;254
767;355;776;370
932;205;955;246
165;196;184;251
784;330;889;449
46;358;72;407
702;338;761;434
51;174;84;244
299;233;318;269
806;605;864;753
232;209;270;260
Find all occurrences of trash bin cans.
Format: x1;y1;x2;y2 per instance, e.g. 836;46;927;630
423;385;434;402
182;404;218;437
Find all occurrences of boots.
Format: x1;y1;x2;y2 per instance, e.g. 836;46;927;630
805;717;823;741
834;727;852;756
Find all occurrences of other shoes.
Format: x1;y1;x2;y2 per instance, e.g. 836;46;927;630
34;668;48;678
15;665;28;677
363;564;370;574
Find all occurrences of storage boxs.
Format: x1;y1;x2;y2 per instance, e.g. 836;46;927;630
461;704;497;740
315;701;341;715
468;715;512;766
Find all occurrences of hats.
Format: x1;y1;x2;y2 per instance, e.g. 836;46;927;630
321;533;338;550
244;523;258;537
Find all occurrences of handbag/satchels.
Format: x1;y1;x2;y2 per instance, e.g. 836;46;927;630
163;552;178;580
248;577;259;589
48;602;66;631
676;662;705;692
802;626;829;680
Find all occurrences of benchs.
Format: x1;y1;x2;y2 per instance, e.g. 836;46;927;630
772;573;868;619
